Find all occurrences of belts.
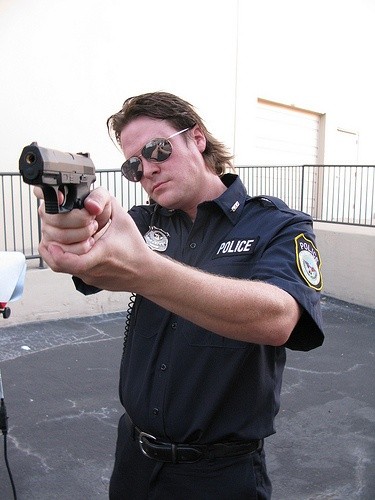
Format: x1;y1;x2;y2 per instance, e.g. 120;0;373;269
125;411;263;463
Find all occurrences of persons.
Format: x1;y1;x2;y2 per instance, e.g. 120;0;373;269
34;91;326;500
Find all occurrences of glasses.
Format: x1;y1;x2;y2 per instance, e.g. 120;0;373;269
121;127;190;182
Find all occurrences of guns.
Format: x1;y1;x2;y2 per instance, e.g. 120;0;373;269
19;141;97;214
